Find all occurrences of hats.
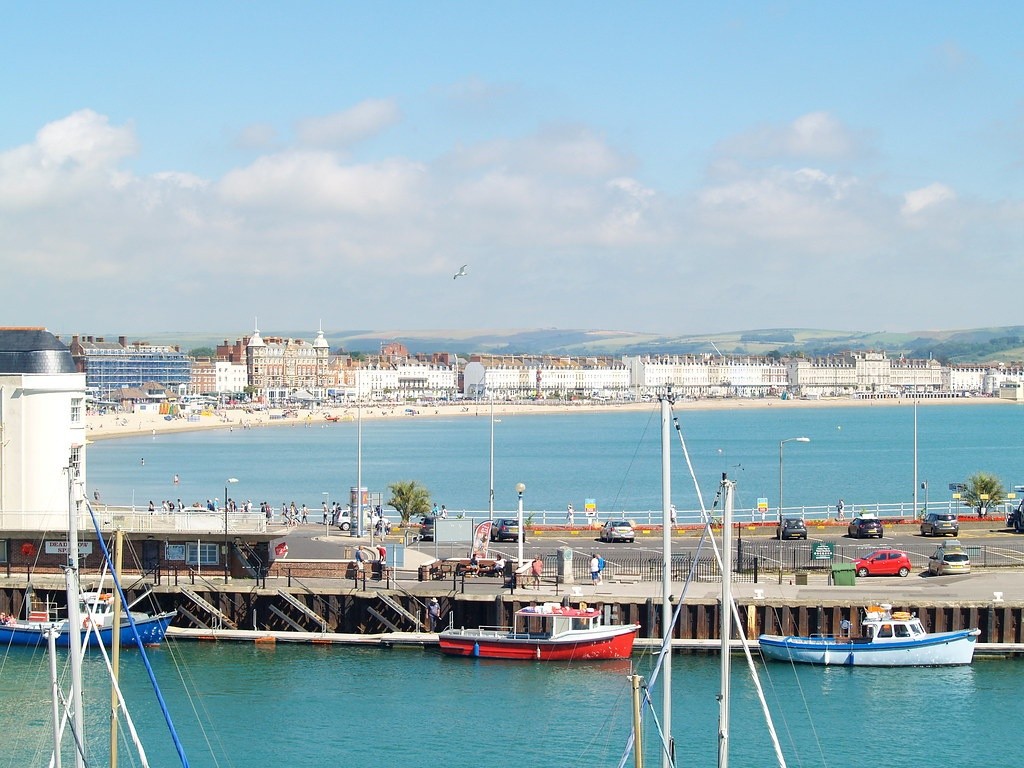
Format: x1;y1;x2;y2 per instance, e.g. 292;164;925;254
431;598;438;602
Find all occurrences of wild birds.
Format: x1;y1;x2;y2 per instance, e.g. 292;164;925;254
454;264;468;280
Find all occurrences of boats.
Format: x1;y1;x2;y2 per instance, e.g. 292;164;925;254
0;582;179;650
756;603;983;668
438;598;642;663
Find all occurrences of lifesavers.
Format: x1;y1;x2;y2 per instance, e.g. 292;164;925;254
84;616;96;628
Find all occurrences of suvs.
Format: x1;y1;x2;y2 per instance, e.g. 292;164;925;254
333;509;391;531
919;512;959;537
416;516;440;542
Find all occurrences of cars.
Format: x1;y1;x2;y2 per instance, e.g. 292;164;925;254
776;517;808;541
850;548;912;578
599;519;635;544
175;506;224;531
928;540;971;577
490;517;526;544
847;516;883;539
1004;497;1024;535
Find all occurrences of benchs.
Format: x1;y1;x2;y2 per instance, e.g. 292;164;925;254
460;559;505;578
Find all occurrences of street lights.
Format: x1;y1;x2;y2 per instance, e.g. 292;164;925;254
224;477;240;585
321;491;329;538
515;482;526;574
778;437;811;585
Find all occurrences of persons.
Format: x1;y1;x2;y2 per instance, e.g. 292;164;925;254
141;457;145;466
93;488;101;501
439;505;448;519
0;613;18;626
670;504;679;529
426;597;440;633
240;499;253;513
355;544;365;580
321;501;350;526
565;503;576;528
148;498;185;516
286;410;331;429
470;554;480;577
431;503;439;516
220;413;262;433
835;499;845;522
174;474;179;484
374;505;392;535
376;545;386;562
260;502;272;525
532;555;543;590
588;553;605;586
205;497;238;513
491;555;507;578
281;501;309;526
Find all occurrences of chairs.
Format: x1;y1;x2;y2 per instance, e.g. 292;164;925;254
432;564;451;581
